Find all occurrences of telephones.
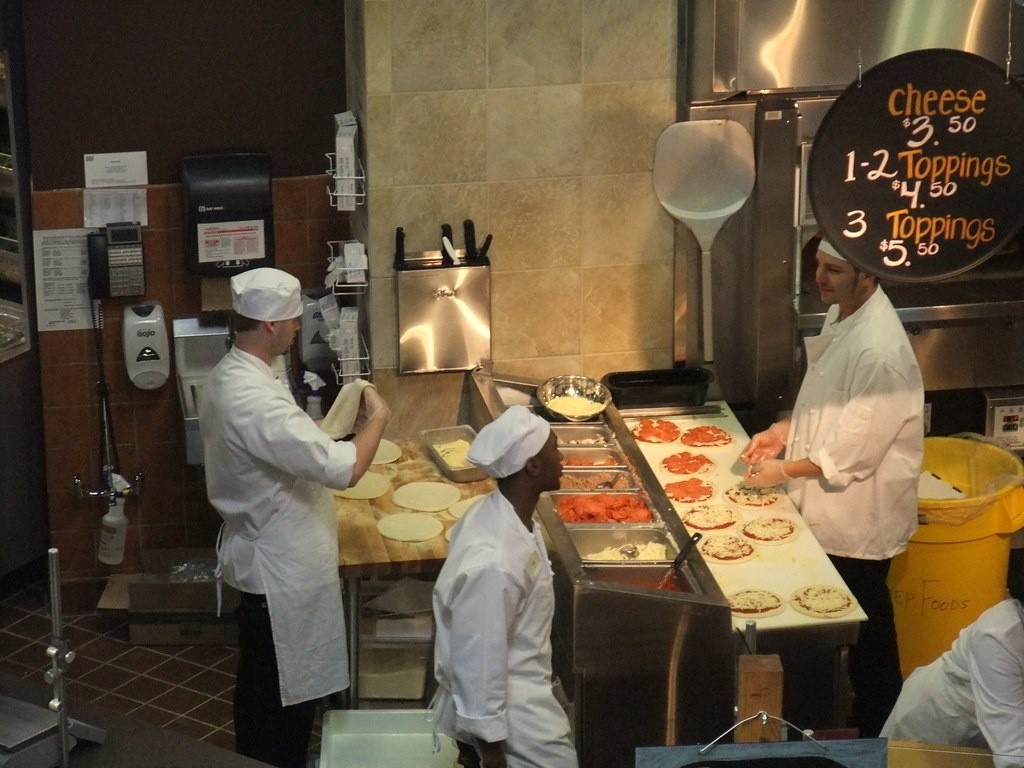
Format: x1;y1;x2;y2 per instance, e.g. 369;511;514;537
87;221;146;301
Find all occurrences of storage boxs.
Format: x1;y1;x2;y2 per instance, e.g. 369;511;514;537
357;592;432;700
92;547;240;614
600;366;715;410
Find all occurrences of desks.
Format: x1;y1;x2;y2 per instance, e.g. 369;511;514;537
332;437;500;712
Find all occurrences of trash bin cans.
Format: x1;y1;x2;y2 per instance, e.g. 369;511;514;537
885;437;1024;681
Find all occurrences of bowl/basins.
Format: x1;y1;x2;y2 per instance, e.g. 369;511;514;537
601;367;714;409
420;424;490;483
537;375;612;421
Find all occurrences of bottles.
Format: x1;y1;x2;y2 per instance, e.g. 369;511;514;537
96;494;132;565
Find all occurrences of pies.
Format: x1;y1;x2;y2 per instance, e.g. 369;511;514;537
319;378;486;543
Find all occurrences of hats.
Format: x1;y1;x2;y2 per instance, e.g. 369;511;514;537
465;404;551;479
818;237;849;263
231;267;304;322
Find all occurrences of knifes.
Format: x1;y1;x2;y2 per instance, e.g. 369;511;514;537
394;221;492;266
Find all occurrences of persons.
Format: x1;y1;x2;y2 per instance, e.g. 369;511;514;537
433;405;582;768
739;231;924;741
198;267;391;767
879;599;1023;768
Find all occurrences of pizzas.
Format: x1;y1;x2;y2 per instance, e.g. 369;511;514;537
631;418;858;619
